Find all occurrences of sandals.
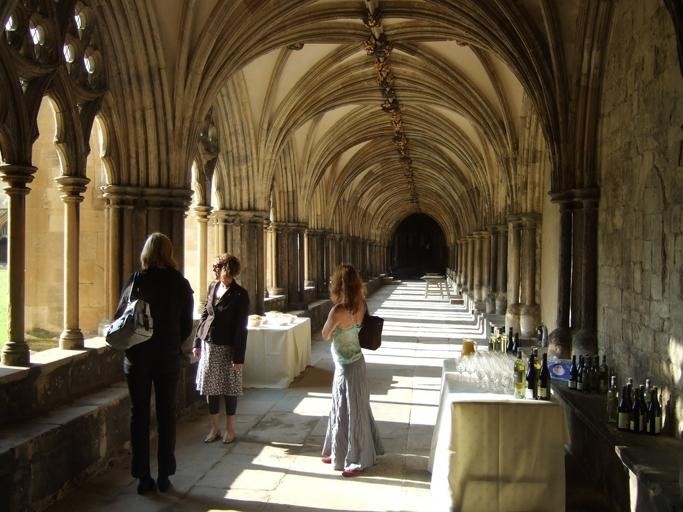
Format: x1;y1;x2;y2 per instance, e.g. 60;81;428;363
205;430;220;442
223;434;235;443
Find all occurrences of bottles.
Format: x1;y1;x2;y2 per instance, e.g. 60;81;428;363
644;379;652;403
577;353;608;392
537;352;550;400
528;348;538;389
568;355;578;389
630;388;644;432
506;327;513;350
640;385;648;431
514;347;526;399
532;357;541;397
617;386;631;430
526;346;536;379
628;378;634;402
647;389;660;435
607;376;619;424
512;333;519;351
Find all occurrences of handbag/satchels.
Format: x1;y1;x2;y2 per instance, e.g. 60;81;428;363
359;298;384;350
106;271;154;351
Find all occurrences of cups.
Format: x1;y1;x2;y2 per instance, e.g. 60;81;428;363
455;352;530;394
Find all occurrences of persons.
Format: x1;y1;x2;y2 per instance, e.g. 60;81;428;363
114;232;194;495
192;253;250;444
322;264;384;478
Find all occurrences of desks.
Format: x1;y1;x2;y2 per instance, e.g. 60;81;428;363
420;275;450;300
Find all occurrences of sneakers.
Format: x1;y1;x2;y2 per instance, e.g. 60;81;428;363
137;480;155;493
157;480;172;491
342;468;362;477
322;457;331;463
